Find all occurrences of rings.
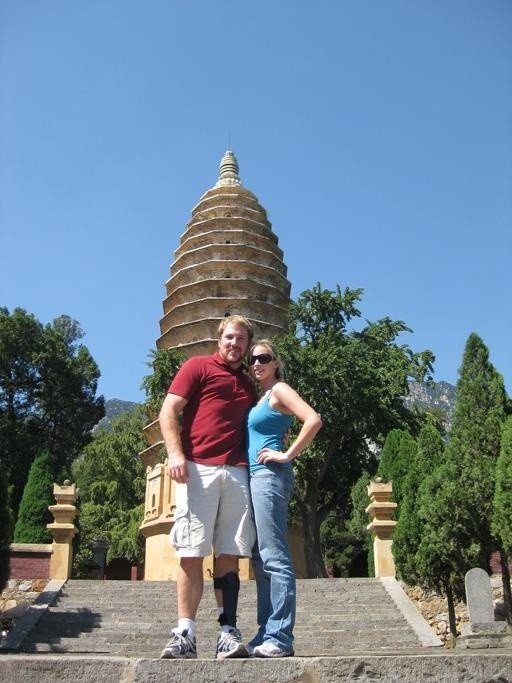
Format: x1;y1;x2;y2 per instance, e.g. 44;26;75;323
265;454;269;457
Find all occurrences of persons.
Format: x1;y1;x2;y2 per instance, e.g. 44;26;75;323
243;338;326;656
156;313;293;658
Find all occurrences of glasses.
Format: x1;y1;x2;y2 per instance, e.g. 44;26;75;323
247;354;276;365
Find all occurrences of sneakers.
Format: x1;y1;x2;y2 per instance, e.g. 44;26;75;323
216;629;249;658
160;630;197;659
254;641;294;658
245;644;255;656
